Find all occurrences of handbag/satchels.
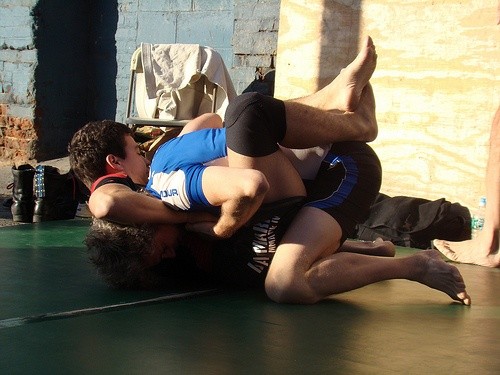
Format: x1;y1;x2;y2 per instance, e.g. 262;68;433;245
357;190;472;250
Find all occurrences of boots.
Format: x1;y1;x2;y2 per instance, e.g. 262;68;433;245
10;164;92;223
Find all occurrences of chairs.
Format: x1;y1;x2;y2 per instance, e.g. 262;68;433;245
125;44;218;127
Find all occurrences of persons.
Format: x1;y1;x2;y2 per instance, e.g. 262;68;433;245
82;113;472;306
432;107;500;268
67;36;396;294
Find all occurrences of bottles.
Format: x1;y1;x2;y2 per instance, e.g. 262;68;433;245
471;197;486;240
